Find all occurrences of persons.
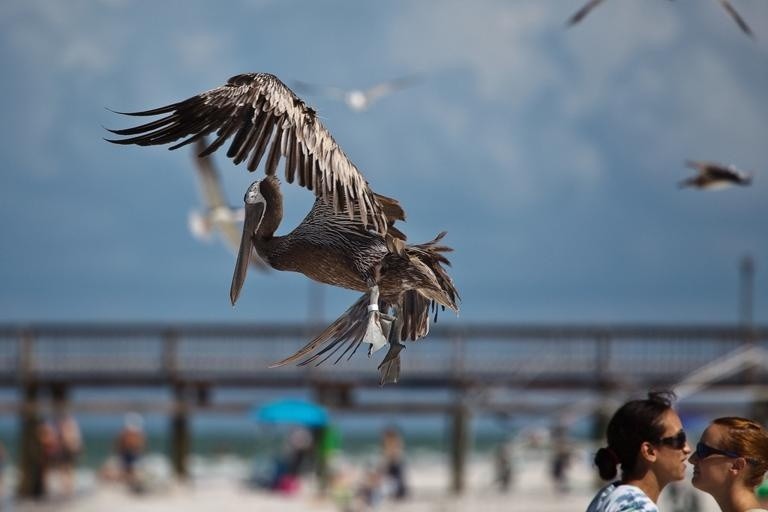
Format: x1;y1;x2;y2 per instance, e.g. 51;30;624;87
485;405;570;489
165;423;410;507
586;391;691;512
688;417;768;512
36;399;152;503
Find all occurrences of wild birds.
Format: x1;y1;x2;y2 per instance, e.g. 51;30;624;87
187;131;272;278
228;173;462;387
102;71;389;239
677;157;757;190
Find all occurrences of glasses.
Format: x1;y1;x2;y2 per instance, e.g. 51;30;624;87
650;428;686;451
696;440;737;461
